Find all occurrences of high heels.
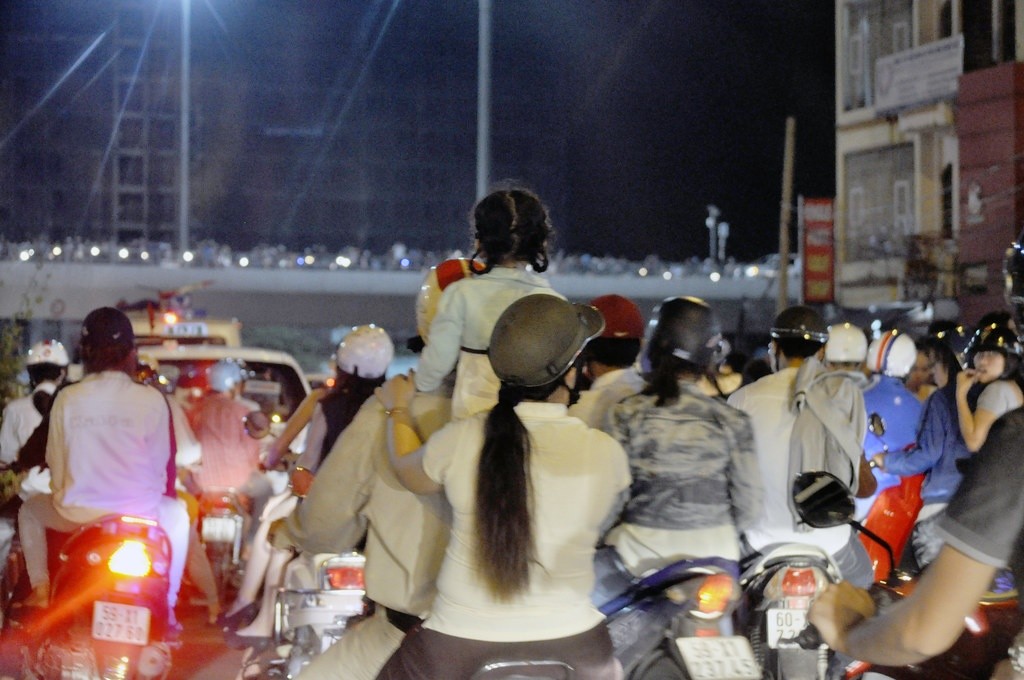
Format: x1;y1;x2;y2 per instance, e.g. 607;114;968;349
223;627;271;665
218;600;258;634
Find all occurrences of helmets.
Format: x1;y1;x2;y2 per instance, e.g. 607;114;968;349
865;329;917;379
26;338;70;368
80;308;131;346
204;359;245;394
593;295;645;339
826;322;868;363
647;295;715;367
488;294;605;388
335;324;394;380
938;326;974;371
769;305;829;343
964;312;1024;360
416;259;488;347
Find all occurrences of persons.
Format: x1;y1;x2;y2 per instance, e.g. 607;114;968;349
826;311;1024;565
186;357;275;561
267;258;632;680
415;189;566;422
565;292;765;610
0;338;70;516
13;307;222;630
217;323;394;651
726;306;874;591
807;405;1024;680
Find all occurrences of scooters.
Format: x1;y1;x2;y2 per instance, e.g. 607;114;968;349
466;468;1024;680
168;486;255;622
231;543;368;679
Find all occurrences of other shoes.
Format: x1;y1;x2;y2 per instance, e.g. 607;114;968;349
207;612;222;628
165;621;184;642
3;603;47;628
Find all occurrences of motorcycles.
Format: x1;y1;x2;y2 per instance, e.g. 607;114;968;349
0;510;179;680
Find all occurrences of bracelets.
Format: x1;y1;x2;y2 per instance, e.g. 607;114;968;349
385;410;401;416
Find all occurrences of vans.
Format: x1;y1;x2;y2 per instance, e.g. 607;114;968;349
137;324;316;468
